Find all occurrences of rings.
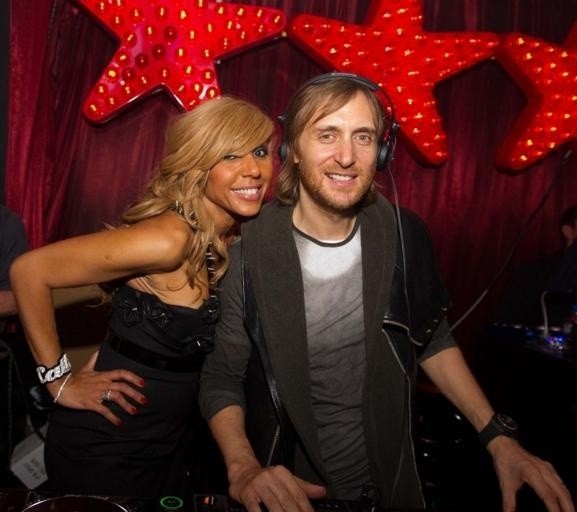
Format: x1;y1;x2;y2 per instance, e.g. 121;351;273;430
101;388;113;399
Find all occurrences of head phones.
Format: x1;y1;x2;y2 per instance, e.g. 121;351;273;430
278;73;400;171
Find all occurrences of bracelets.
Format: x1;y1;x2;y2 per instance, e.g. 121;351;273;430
36;352;75;381
53;372;73;403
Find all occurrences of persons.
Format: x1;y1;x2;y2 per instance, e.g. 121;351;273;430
200;74;576;510
525;202;575;326
2;209;31;297
7;94;281;511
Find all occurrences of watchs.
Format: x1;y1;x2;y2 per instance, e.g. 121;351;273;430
479;409;519;445
